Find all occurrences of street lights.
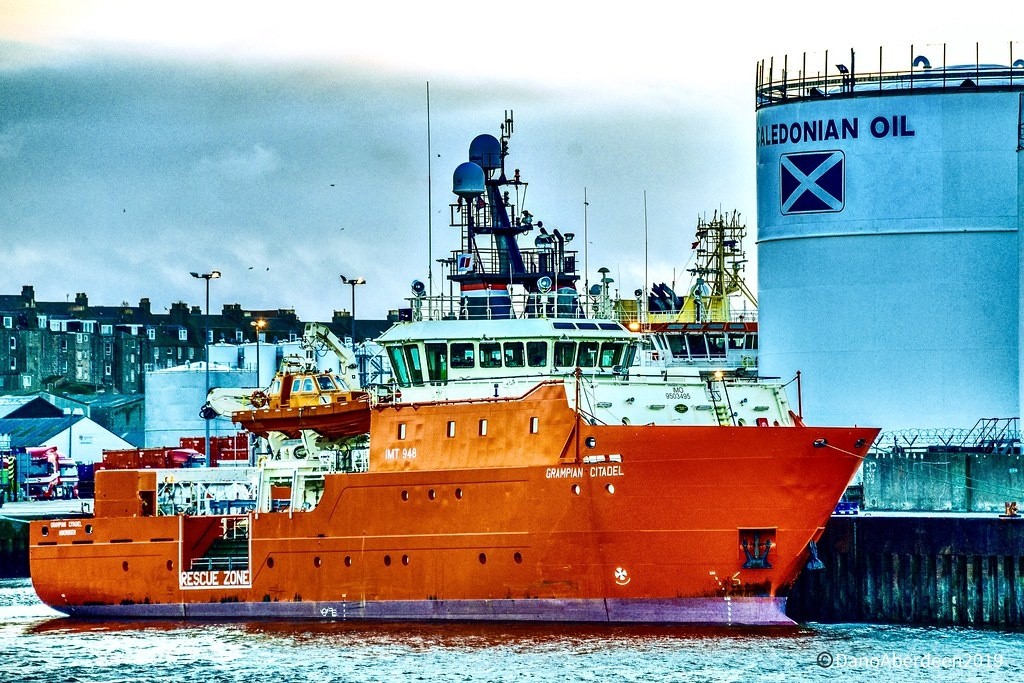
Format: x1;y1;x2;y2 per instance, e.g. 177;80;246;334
338;272;369;355
249;318;269;389
189;268;222;466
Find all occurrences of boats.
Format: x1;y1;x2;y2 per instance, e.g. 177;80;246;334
371;108;810;426
28;367;880;643
225;353;373;441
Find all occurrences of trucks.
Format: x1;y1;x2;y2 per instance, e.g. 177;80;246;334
56;456;79;497
100;446;207;471
23;446;61;499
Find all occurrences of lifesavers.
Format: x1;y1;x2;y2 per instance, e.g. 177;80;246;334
251;390;268;408
